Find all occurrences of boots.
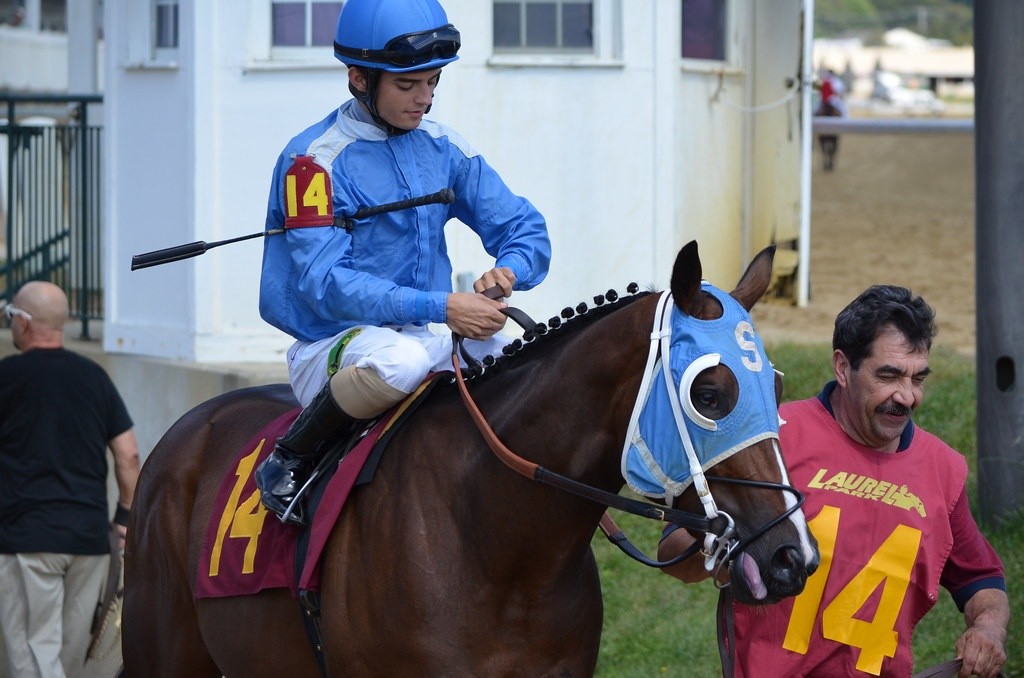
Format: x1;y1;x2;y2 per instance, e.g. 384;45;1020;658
253;369;379;525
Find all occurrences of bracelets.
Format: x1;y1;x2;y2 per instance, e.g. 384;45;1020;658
114;504;129;526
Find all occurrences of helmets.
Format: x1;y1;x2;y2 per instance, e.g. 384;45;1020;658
333;0;462;74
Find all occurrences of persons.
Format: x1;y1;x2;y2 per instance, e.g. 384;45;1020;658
812;71;846;115
0;281;140;677
254;1;553;524
656;284;1010;677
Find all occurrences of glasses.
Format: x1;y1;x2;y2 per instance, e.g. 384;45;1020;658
383;23;461;68
5;303;33;321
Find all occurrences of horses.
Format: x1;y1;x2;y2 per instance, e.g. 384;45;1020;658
116;238;822;678
